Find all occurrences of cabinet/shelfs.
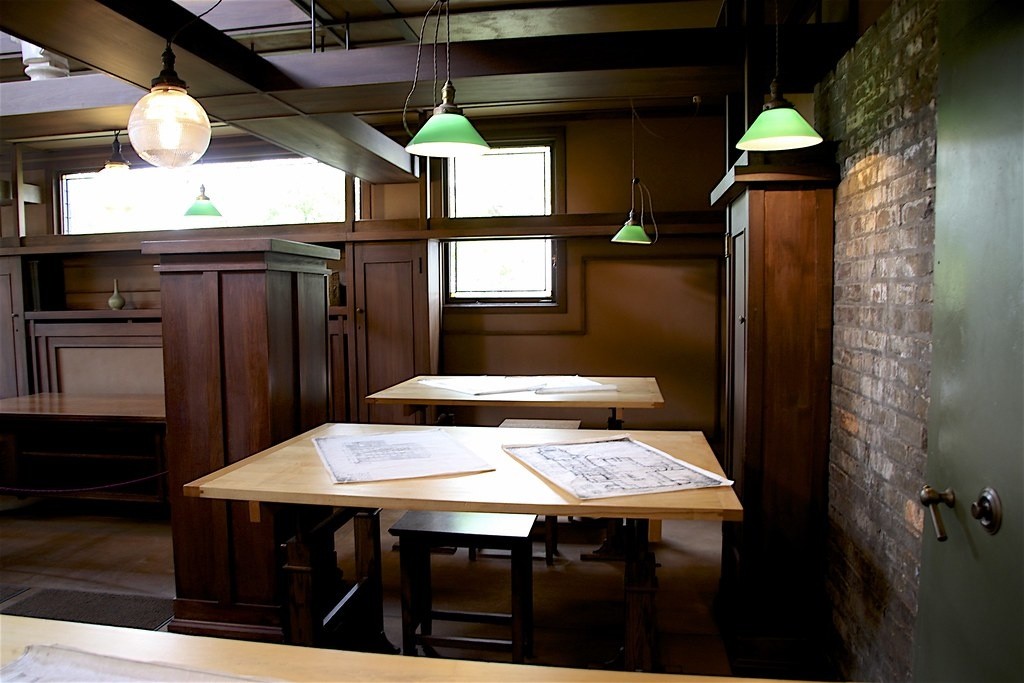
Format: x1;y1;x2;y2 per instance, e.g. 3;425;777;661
141;239;341;644
350;242;426;425
0;255;29;400
711;163;830;574
0;393;168;503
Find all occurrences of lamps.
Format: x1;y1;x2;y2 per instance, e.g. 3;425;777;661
612;112;651;244
127;36;211;167
405;0;491;157
736;8;823;153
185;184;222;216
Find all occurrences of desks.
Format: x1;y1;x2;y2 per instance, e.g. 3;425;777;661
183;422;743;675
365;374;665;540
0;614;791;682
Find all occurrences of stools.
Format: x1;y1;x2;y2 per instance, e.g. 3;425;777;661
467;418;580;567
387;509;538;665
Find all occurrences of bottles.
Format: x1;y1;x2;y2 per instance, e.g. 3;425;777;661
108;279;126;310
